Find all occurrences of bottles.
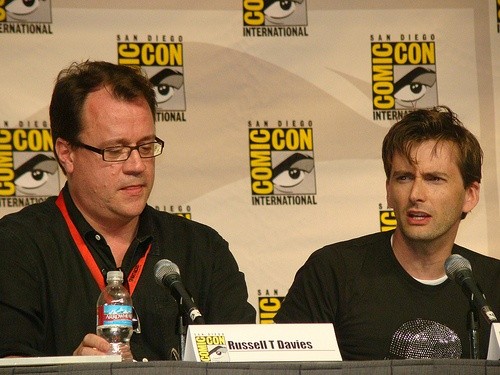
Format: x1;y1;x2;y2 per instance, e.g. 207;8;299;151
97;270;133;363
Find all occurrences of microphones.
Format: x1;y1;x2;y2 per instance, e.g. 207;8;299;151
154;258;205;325
444;254;497;326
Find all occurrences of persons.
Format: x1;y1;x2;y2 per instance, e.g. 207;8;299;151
1;59;257;358
273;99;498;362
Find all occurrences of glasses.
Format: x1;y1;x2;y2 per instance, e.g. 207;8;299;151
60;136;164;161
